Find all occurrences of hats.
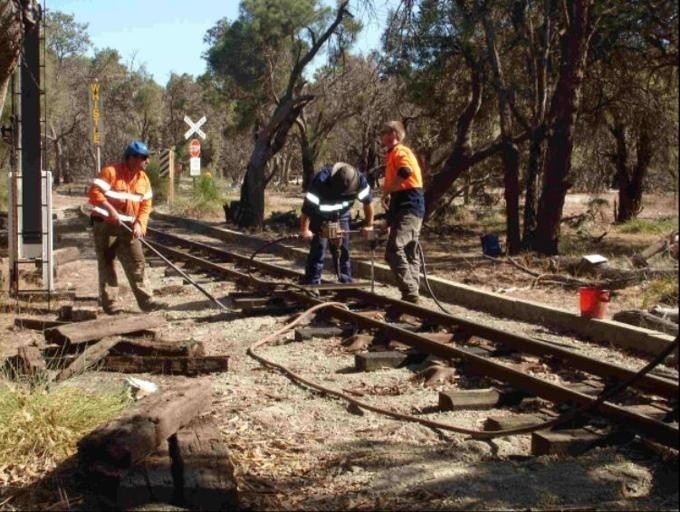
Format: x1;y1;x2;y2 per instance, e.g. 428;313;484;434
330;162;359;196
126;142;156;155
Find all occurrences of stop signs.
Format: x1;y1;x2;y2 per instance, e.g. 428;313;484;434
190;139;201;157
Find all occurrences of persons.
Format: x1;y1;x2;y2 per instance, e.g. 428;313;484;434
88;141;167;315
300;162;374;297
381;120;425;304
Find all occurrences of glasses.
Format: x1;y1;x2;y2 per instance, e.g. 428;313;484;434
132;154;146;160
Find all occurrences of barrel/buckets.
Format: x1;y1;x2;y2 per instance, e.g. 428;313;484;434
577;286;611;318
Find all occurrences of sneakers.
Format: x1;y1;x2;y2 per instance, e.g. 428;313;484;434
142;301;168;312
